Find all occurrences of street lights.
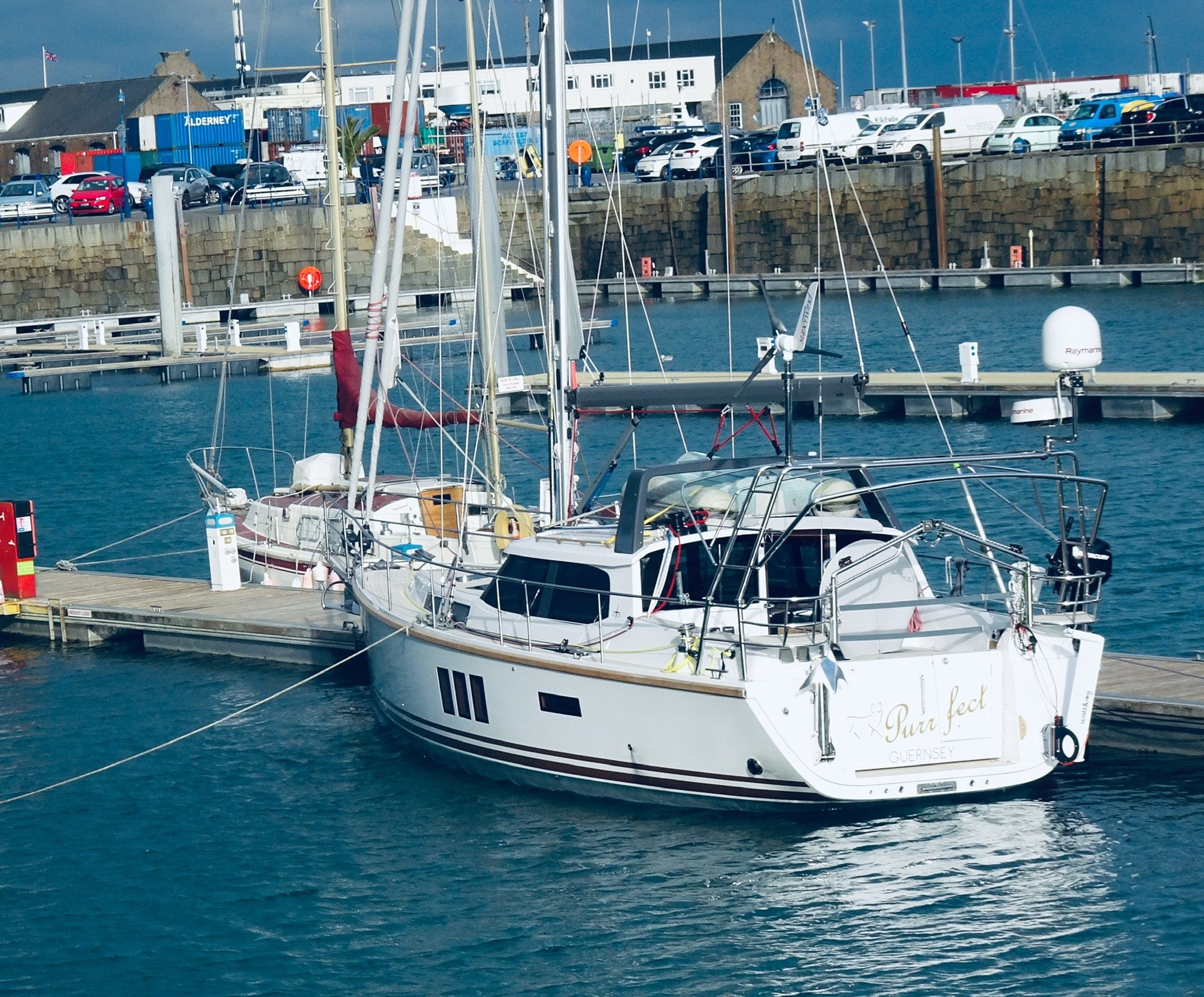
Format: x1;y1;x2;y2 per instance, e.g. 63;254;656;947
862;20;877;110
953;35;966;97
117;88;130;218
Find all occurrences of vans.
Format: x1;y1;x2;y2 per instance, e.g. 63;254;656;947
876;104;1005;161
1057;91;1182;150
776;115;836;169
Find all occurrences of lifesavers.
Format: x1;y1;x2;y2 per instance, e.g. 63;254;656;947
493;505;532;548
299;266;322;291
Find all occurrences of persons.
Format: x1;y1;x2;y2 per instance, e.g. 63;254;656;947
358;158;380;204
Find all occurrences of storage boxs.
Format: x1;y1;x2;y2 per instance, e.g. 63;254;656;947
61;100;543;185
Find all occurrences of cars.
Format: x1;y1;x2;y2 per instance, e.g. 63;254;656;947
987;112;1067;154
46;170;147;214
65;175;130;217
227;161;294;204
140;166;212;212
378;153;437;194
634;139;687;182
1119;92;1204;147
669;133;752;181
829;121;917;165
712;130;782;176
0;172;58;222
367;153;456;190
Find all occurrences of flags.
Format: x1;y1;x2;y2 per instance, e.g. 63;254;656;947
44;48;57;62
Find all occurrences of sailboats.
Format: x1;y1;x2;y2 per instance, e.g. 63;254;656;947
185;0;1115;822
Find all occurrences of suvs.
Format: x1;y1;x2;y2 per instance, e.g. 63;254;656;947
622;131;720;174
138;162;234;204
